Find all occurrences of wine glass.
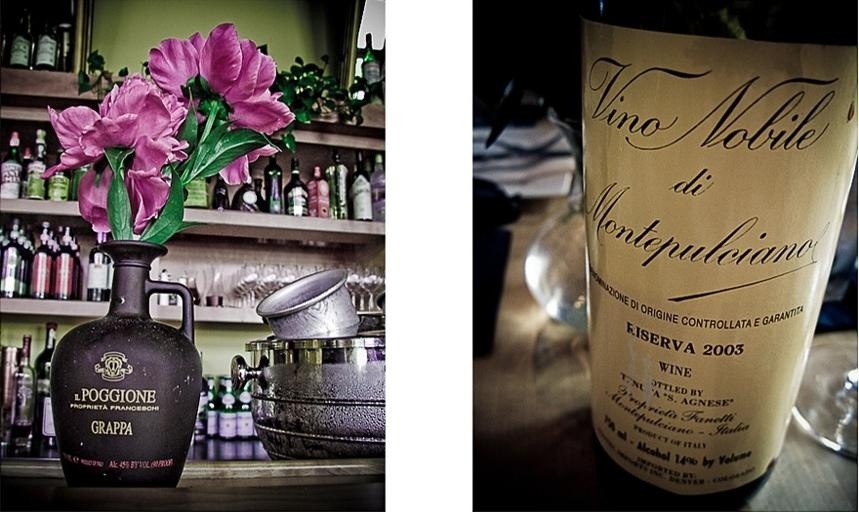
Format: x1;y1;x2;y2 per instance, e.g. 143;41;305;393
233;258;386;311
788;340;858;459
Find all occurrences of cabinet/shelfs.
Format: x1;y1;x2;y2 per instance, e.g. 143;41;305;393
0;54;385;327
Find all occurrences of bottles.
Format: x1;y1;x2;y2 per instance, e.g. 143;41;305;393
0;318;260;456
0;2;74;73
578;2;859;502
0;214;201;309
0;126;386;222
360;30;386;105
208;266;229;309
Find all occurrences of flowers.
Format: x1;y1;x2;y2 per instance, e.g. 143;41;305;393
41;23;362;244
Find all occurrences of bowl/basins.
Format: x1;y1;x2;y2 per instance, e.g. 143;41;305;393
257;268;360;339
